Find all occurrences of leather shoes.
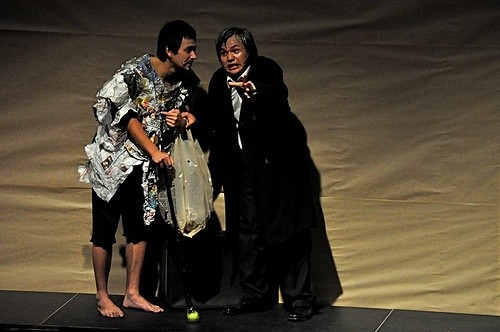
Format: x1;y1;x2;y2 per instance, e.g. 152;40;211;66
222;295;275;315
288;305;314;320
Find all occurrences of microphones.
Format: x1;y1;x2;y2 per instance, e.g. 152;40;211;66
177;54;185;64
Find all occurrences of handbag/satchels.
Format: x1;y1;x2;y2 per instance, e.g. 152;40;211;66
158;118;213;238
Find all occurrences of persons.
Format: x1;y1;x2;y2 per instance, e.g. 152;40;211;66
87;19;197;318
180;27;320;322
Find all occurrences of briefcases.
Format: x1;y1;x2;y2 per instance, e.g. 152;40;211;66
159;231;243;310
